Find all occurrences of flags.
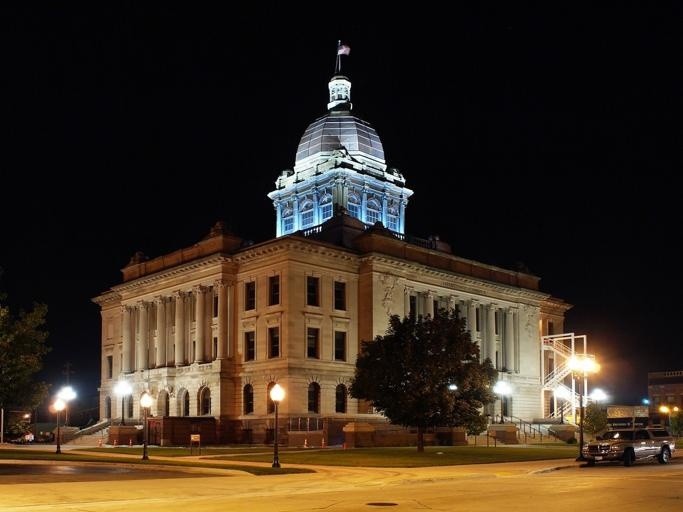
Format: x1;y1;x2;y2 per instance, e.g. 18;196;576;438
337;44;350;55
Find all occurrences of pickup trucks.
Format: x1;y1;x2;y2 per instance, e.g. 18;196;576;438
587;425;676;466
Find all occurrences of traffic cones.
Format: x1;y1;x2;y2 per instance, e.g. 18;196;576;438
301;436;310;448
93;436;134;451
320;437;326;448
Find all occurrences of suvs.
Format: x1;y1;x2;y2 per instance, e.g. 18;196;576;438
646;424;669;430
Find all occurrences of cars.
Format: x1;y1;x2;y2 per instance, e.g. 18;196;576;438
9;430;55;448
580;439;591;458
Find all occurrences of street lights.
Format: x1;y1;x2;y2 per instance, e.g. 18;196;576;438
267;383;287;471
587;386;606;412
113;379;133;425
59;385;77;427
51;397;67;455
660;406;680;435
567;357;600;462
137;394;154;460
490;380;510;424
